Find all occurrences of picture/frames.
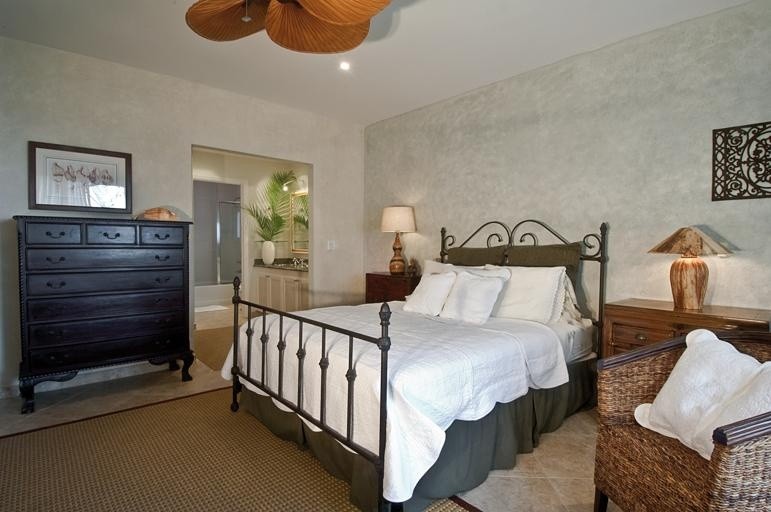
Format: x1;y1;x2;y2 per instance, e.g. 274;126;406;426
28;141;133;214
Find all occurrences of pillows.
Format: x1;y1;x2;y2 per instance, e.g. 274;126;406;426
551;266;566;322
403;271;457;316
507;242;582;289
438;271;504;325
446;245;505;266
634;328;771;462
405;259;511;302
485;264;563;324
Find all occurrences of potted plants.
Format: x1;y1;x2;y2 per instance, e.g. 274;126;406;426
232;169;297;265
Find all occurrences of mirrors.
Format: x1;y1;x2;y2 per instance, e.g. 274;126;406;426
289;192;308;254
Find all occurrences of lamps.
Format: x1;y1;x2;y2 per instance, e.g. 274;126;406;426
647;227;734;310
185;0;393;54
381;207;415;274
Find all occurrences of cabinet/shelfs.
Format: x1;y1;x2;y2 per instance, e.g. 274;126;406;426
12;214;193;414
256;272;280;313
601;298;771;359
281;275;308;313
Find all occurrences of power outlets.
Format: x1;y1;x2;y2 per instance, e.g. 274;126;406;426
327;239;336;251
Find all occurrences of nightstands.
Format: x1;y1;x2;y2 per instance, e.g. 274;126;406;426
365;272;421;303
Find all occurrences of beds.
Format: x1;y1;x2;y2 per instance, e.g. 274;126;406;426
221;219;610;512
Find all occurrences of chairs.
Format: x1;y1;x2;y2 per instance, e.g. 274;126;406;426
594;330;771;512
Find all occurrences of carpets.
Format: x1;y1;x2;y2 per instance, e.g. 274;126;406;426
195;324;242;370
0;385;483;512
194;304;229;312
